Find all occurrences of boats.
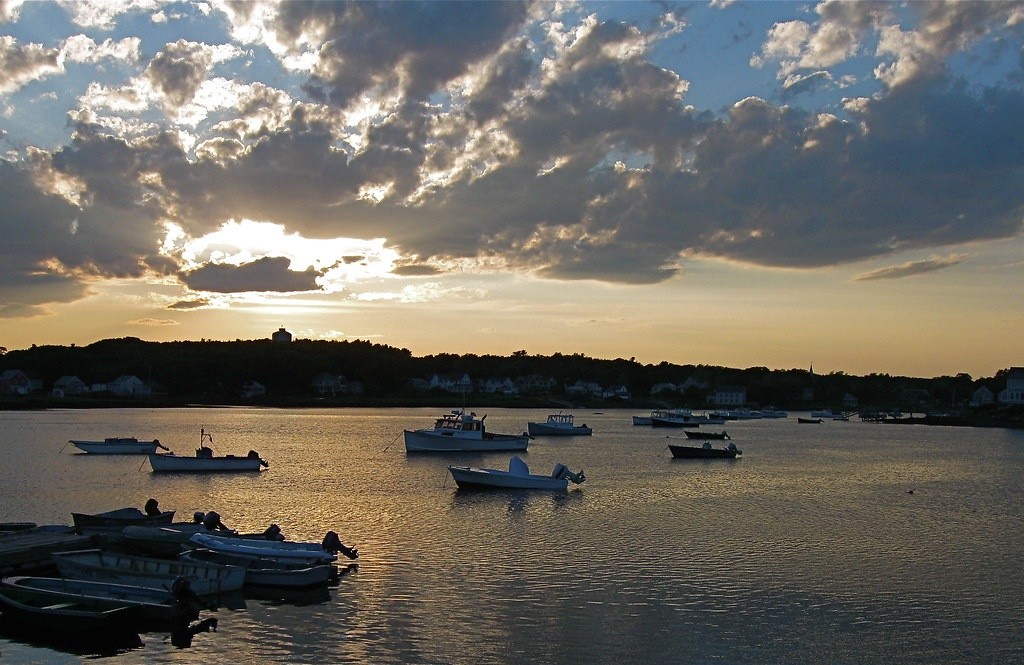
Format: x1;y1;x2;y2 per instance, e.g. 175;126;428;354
49;541;249;596
797;417;824;424
0;573;199;638
632;406;788;425
405;386;536;453
669;441;743;458
858;412;886;418
120;511;284;559
527;413;591;437
177;547;333;587
0;521;76;534
652;418;700;427
888;412;904;417
812;409;843;417
684;430;732;441
149;427;269;474
69;499;176;537
189;531;358;565
448;458;586;491
69;437;169;455
